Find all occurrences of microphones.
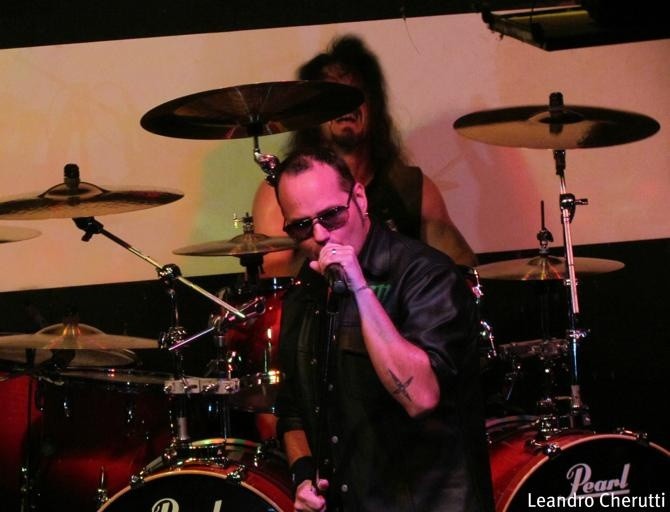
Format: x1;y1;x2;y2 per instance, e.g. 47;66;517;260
325;259;349;292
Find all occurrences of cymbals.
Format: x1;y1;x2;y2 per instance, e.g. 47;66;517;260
453;105;661;150
1;320;163;349
1;349;138;365
140;80;365;140
471;256;624;281
0;182;185;220
172;231;299;256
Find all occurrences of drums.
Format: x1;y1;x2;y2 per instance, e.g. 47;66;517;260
485;414;670;512
98;437;295;512
211;274;295;413
30;373;212;510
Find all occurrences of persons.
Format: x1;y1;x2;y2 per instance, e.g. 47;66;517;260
271;145;496;512
249;32;478;287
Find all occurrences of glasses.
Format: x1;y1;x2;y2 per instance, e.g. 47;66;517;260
283;183;353;242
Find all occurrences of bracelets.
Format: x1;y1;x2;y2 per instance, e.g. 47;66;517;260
288;457;317;497
